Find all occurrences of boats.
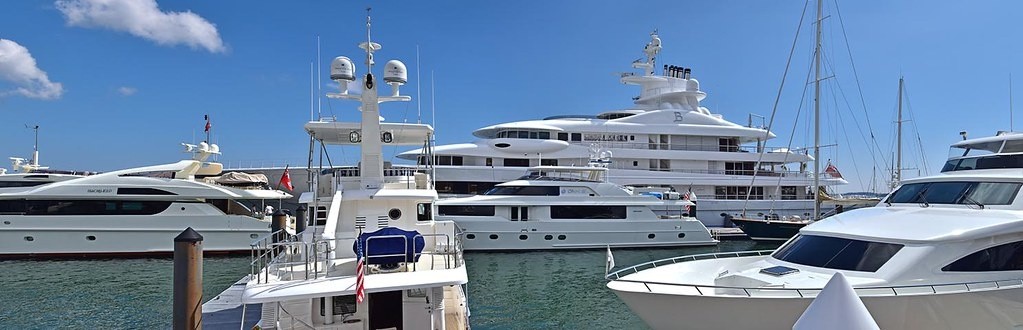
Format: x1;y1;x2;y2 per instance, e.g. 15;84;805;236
202;4;472;330
0;114;295;258
601;0;1023;329
396;30;848;253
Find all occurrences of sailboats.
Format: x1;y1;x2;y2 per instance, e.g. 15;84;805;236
731;0;933;241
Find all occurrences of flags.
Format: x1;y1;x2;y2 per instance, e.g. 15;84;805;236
683;184;691;213
204;120;211;132
356;232;365;304
280;168;293;192
823;161;844;180
